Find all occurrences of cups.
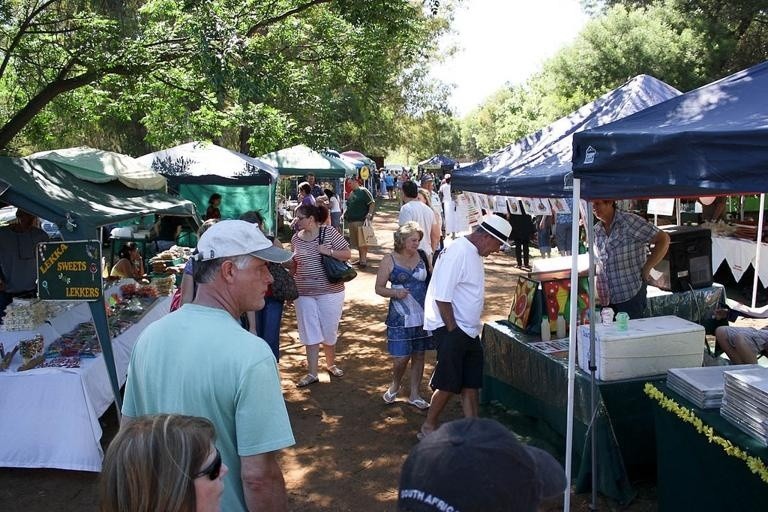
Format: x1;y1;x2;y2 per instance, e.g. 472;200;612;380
601;307;615;326
615;311;631;331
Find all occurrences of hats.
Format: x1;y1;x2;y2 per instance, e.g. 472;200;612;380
477;214;513;248
197;220;295;263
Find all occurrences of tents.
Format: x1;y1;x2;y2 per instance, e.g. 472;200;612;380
253;141;377;199
418;155;454;180
136;139;278;238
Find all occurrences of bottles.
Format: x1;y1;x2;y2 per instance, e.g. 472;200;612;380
555;312;567;338
540;315;551;342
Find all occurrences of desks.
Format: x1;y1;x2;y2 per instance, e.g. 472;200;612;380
645;279;725;358
108;235;160;273
484;312;735;511
709;232;768;319
0;289;172;474
640;377;768;511
148;254;191;286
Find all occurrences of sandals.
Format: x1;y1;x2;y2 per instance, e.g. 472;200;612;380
351;261;367;269
383;385;401;403
407;397;431;410
296;373;320;387
326;364;344;376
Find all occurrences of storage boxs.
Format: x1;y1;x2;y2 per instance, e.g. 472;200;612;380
576;314;707;382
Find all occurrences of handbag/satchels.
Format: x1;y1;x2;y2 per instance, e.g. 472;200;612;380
321;254;357;283
357;219;380;247
267;262;298;300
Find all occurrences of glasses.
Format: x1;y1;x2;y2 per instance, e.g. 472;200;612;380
298;216;308;221
192;446;222;481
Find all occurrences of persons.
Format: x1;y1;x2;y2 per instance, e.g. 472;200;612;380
110;174;374;387
373;170;572;441
593;201;671;322
119;220;295;511
0;208;49;325
716;325;768;365
98;413;228;512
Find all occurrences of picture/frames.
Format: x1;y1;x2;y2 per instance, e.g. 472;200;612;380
508;268;591;336
